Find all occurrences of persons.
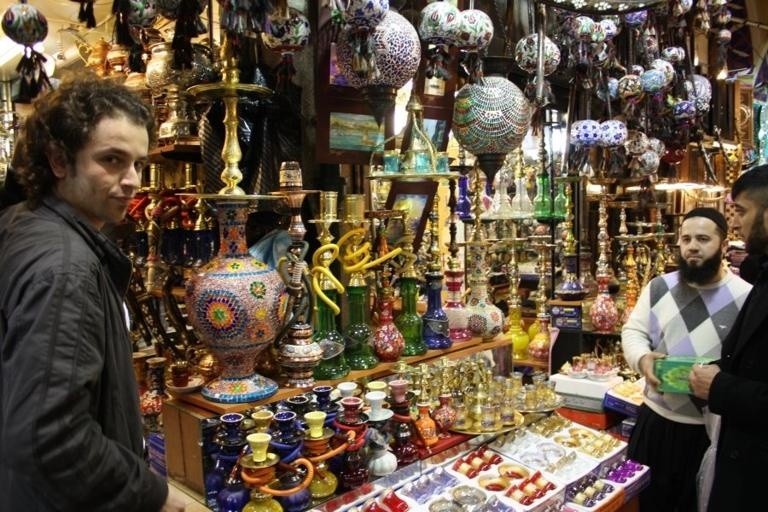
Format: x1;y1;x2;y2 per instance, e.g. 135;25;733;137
687;165;765;512
0;74;188;512
621;205;752;511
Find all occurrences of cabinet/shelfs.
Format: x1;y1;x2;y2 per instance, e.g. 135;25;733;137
2;1;768;512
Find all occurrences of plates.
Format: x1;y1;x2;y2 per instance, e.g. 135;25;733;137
429;485;486;512
479;462;531;491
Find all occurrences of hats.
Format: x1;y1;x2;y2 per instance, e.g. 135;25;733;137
683;208;729;232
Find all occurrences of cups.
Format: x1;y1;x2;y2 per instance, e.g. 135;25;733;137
506;470;557;506
453;445;503;478
480;370;557;428
487;495;512;512
572;353;590;372
531;411;643;508
361;465;458;512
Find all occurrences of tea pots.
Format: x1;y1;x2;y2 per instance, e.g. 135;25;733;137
463;382;489;419
